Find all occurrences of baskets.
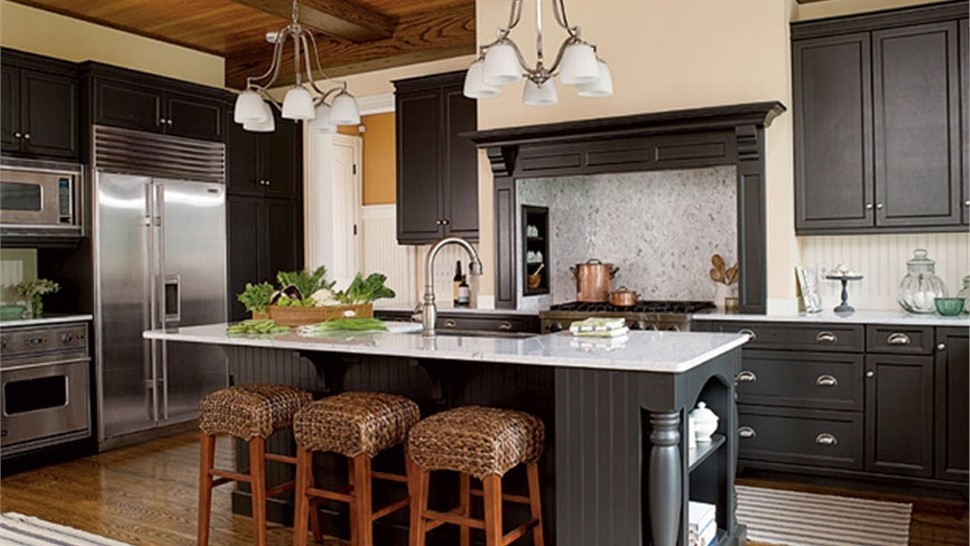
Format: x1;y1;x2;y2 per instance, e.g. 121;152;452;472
253;303;373;327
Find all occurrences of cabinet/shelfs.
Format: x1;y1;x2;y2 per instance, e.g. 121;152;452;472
78;58;305;325
789;0;970;236
641;346;747;546
0;45;79;163
690;318;970;493
520;204;551;296
390;71;481;248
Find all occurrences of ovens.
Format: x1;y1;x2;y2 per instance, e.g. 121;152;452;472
0;325;92;462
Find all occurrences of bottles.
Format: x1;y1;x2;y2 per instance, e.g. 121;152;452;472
956;275;970;314
457;275;469;305
896;249;948;314
453;260;462;308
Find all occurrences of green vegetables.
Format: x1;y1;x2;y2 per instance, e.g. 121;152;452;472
236;265;395;314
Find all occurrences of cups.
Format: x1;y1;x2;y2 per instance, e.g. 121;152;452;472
528;275;542;288
724;298;739;314
691;402;719;442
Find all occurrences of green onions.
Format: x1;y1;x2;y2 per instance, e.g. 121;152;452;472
297;318;388;332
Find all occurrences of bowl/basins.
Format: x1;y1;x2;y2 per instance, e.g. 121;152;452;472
934;297;966;316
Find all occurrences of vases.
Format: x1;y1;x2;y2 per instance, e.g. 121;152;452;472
31;296;44;318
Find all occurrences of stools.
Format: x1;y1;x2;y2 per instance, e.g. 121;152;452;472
408;405;545;546
197;382;311;546
291;391;422;546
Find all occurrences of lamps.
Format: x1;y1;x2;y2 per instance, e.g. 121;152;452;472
463;0;613;106
234;0;361;132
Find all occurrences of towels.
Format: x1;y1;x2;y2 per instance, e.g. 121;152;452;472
568;316;630;338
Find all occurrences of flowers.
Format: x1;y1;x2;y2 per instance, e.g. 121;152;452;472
16;278;59;298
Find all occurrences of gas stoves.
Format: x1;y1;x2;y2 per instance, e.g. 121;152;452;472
539;299;717;333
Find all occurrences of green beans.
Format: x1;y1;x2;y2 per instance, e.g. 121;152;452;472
225;316;291;333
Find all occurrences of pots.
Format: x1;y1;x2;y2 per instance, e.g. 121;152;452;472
570;258;620;303
608;291;641;306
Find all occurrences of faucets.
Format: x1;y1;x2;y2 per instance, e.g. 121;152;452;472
420;236;483;338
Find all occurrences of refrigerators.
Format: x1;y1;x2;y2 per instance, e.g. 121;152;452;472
90;124;230;453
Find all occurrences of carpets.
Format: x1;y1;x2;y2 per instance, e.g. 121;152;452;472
734;484;912;546
0;509;136;546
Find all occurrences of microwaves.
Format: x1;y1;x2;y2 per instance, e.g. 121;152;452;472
0;150;85;238
